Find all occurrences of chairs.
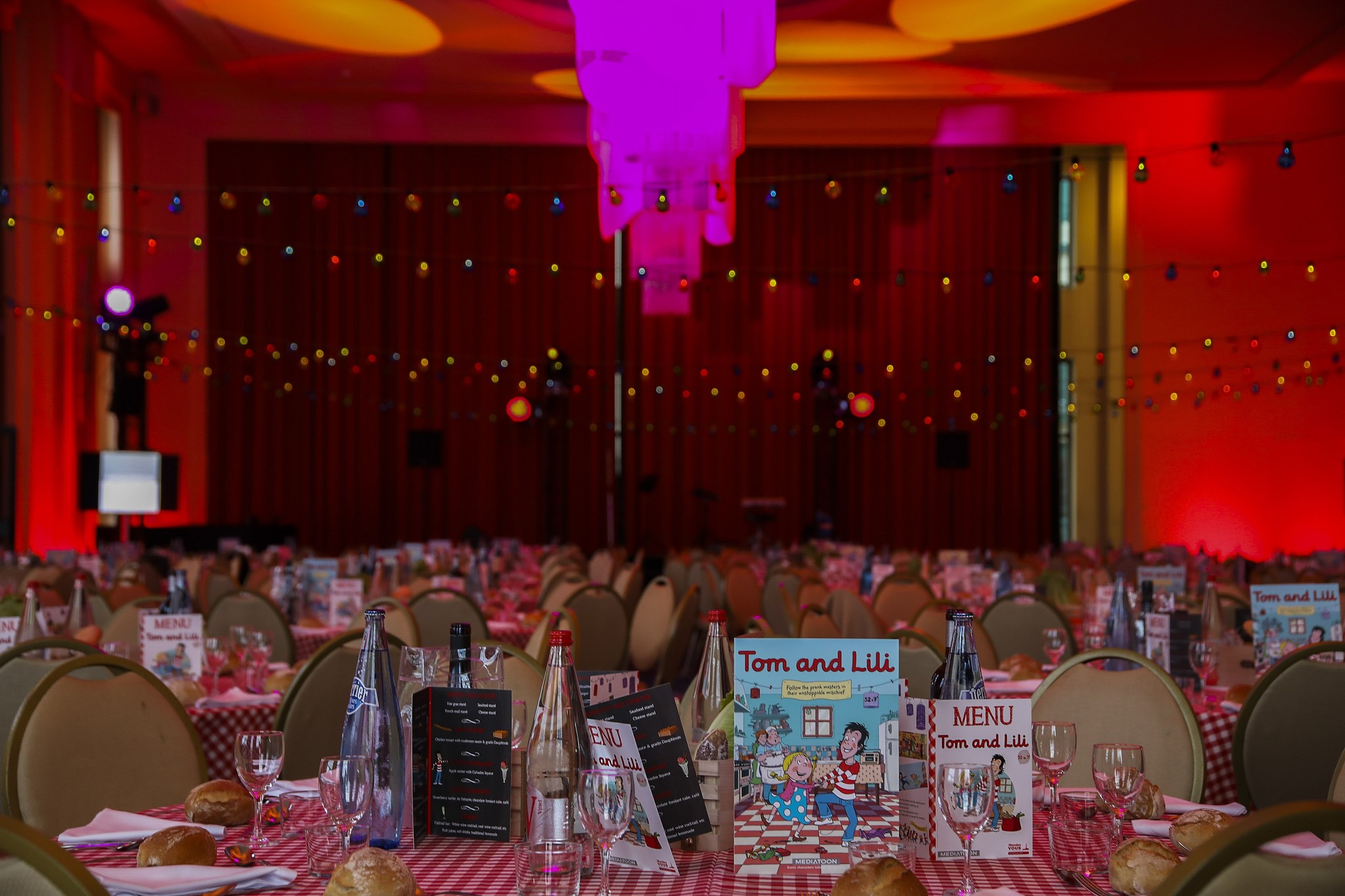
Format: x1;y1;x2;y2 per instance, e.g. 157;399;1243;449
2;533;1341;896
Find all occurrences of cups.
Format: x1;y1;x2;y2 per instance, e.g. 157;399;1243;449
278;789;334;838
307;824;370;879
1031;776;1113;881
847;839;917;874
515;841;581;896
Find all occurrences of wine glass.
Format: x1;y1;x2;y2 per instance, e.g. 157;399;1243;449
196;625;274;712
1031;720;1076;829
1040;624;1067;670
318;754;374;861
1091;741;1145;845
1193;642;1217;706
937;761;995;894
232;728;284;848
577;766;635;896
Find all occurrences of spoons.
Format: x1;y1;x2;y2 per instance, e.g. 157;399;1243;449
112;882;238;896
222;844;271;867
55;837;145;852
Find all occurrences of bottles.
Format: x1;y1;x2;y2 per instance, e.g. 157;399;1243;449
1200;586;1222;672
693;610;734;756
1106;574;1158;670
338;603;405;851
851;545;1247;624
526;628;595;877
5;539;505;685
928;606;987;701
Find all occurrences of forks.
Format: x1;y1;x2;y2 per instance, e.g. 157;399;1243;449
1072;870;1119;896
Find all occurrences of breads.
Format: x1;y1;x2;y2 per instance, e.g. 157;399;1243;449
322;846;416;896
186;778;253;828
263;669;298;696
136;826;217;867
1095;767;1165;819
1225;684;1254;703
831;856;927;896
1170;809;1239;855
997;653;1042;683
160;678;208;707
1108;838;1181;894
